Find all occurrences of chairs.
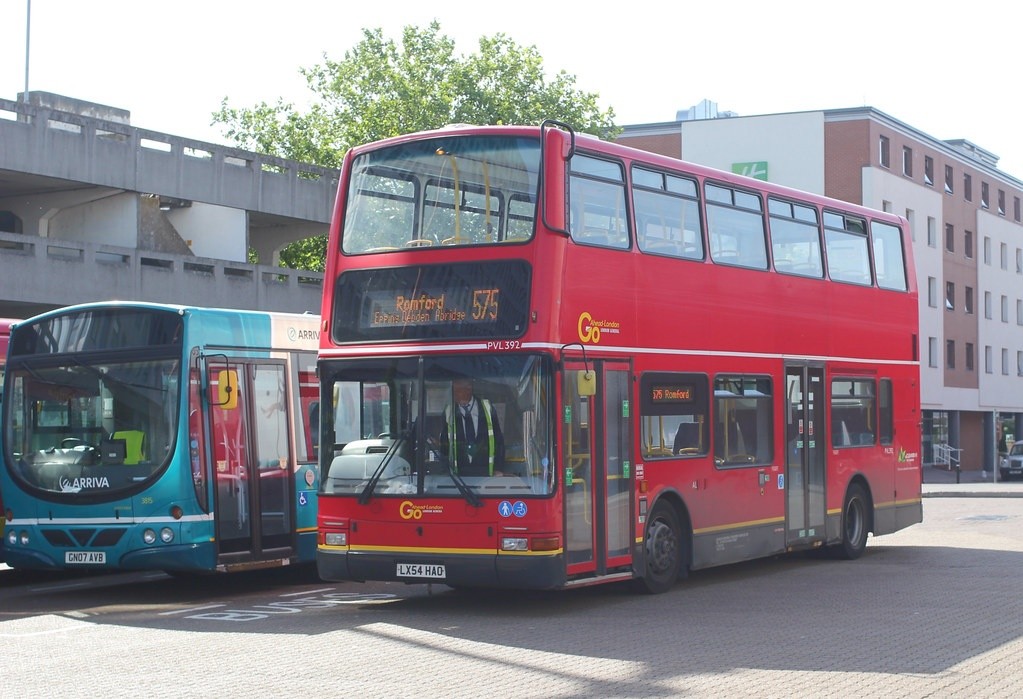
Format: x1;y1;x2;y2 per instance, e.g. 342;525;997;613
365;231;901;287
573;401;882;457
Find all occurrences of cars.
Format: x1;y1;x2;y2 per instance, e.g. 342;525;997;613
932;425;948;444
1000;440;1023;479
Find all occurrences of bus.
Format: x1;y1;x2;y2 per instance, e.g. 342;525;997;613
314;118;923;592
0;303;390;583
0;316;116;478
181;368;388;489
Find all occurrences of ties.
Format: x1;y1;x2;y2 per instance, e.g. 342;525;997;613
462;404;476;442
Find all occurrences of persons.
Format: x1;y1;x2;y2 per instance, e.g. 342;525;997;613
999;434;1008;459
441;373;505;477
